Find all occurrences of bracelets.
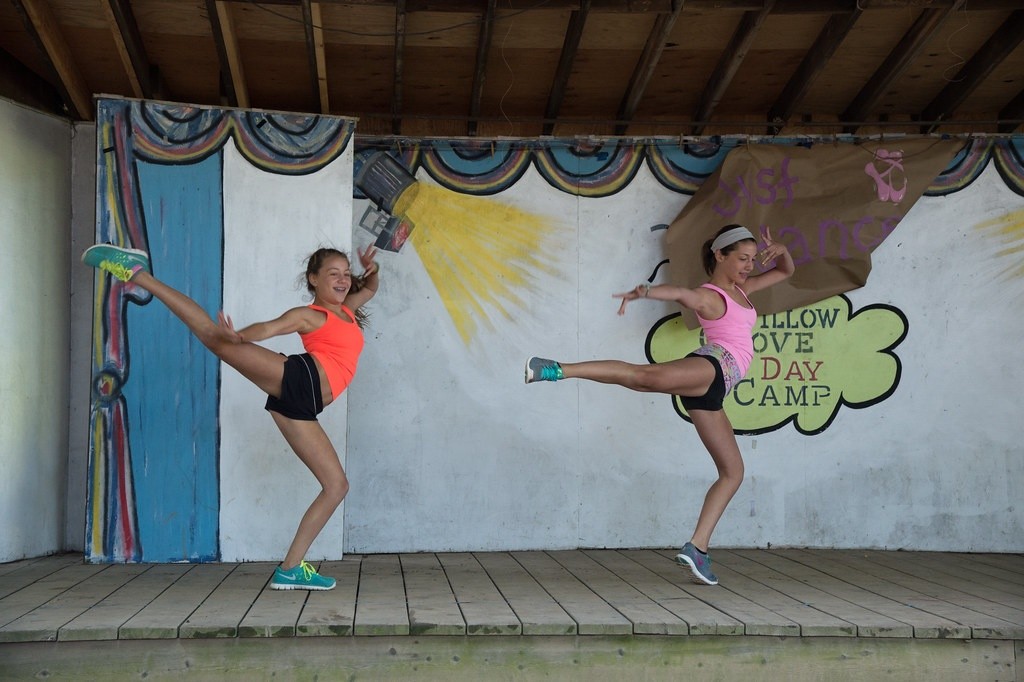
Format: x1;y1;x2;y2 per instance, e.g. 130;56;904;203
645;285;650;297
237;334;243;341
372;262;380;274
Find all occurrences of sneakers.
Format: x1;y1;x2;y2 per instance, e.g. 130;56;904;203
81;244;150;283
673;542;720;586
524;355;564;383
269;559;337;590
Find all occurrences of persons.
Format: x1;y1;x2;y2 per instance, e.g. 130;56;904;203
80;243;380;590
525;224;796;585
